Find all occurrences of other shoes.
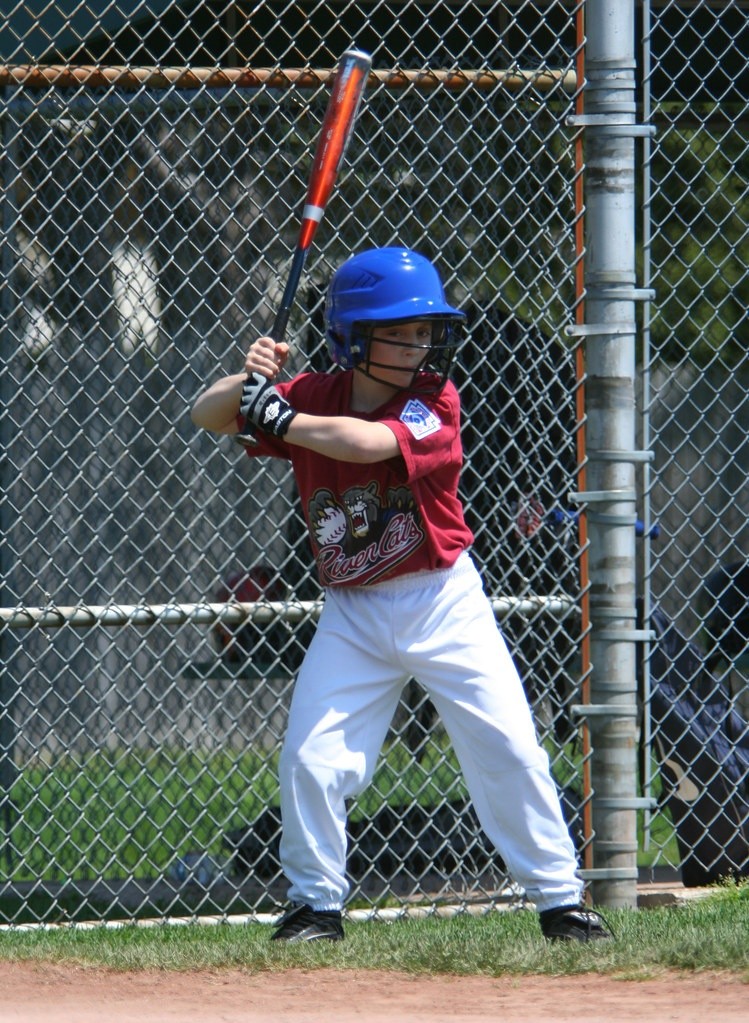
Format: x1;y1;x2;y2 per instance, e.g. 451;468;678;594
537;905;618;948
270;903;345;948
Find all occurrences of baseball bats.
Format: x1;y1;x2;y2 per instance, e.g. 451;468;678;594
231;48;372;449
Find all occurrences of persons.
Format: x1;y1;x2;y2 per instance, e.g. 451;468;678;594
190;248;607;942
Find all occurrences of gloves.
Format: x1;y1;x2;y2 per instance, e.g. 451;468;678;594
238;371;298;442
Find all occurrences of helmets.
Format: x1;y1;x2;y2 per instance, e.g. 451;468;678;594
322;247;468;395
